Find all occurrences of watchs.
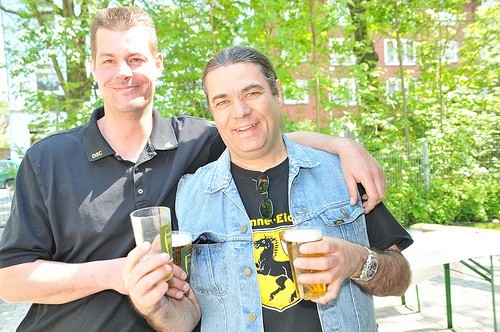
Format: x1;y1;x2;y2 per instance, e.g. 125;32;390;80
349;247;378;282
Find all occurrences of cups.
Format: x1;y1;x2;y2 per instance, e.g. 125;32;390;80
283;227;327;300
172;231;193;285
130;207;174;281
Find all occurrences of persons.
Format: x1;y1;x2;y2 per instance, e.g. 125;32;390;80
0;7;386;332
123;46;412;332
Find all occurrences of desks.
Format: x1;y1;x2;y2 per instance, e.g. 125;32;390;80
401;227;500;332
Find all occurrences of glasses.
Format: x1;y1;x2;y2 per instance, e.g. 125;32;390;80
256;174;275;224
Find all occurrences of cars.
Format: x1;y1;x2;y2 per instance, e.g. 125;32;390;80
0;159;22;192
